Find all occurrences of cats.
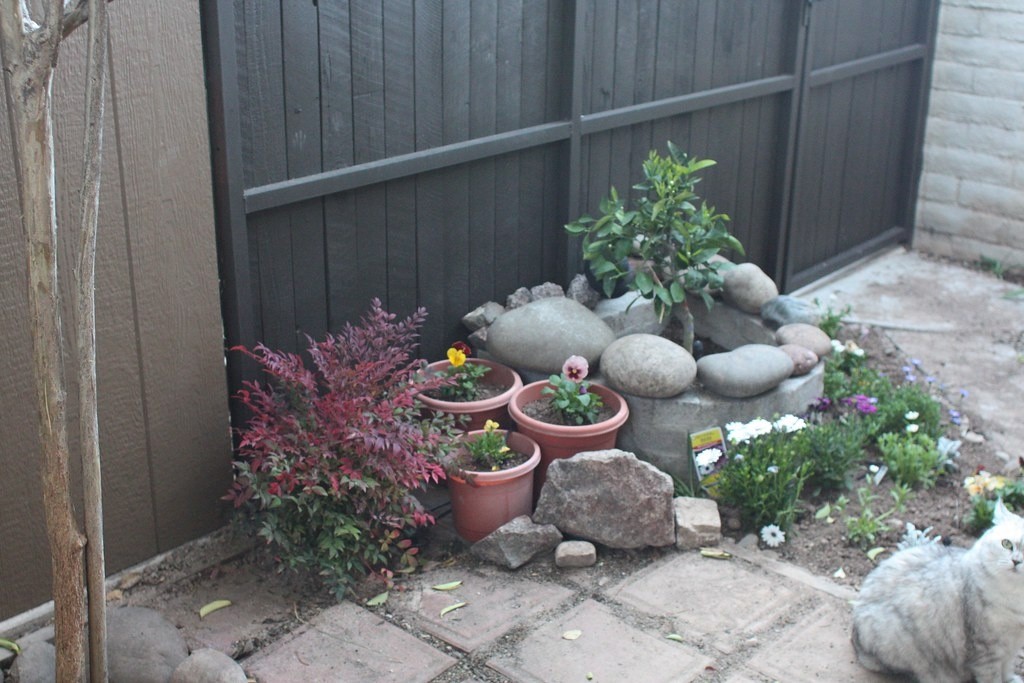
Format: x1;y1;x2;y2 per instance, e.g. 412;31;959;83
851;493;1024;683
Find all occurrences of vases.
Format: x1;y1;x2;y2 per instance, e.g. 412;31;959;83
408;357;523;432
508;380;630;494
445;429;542;544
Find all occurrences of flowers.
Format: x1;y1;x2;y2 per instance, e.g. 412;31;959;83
429;341;491;402
541;354;604;423
463;419;520;473
